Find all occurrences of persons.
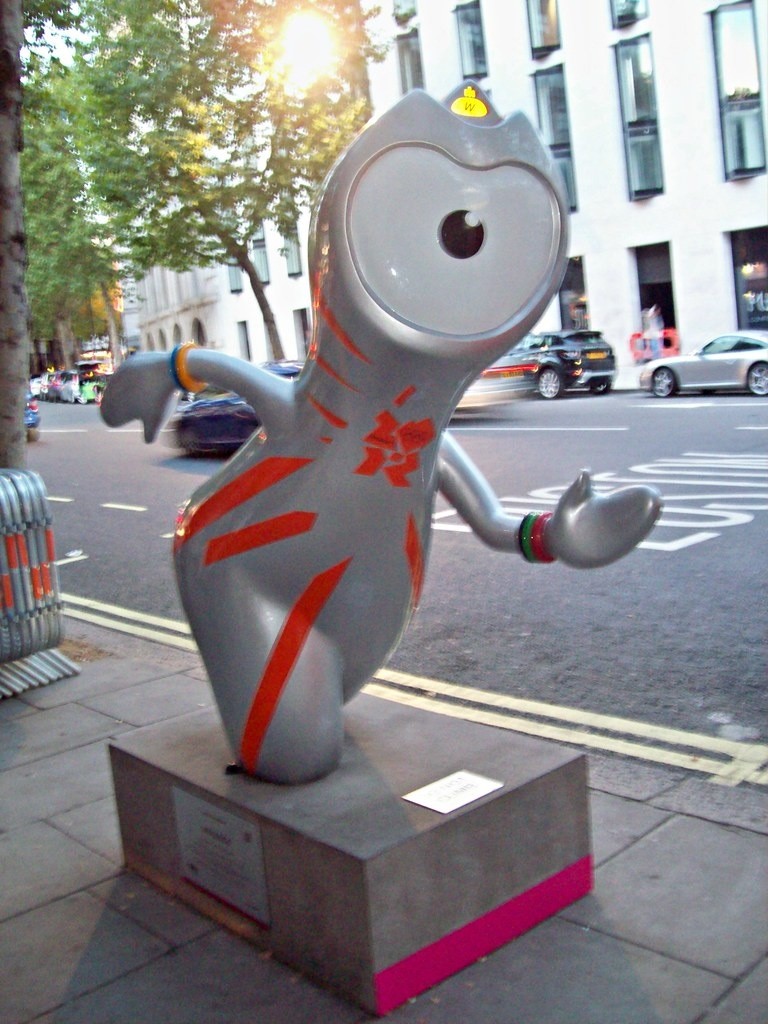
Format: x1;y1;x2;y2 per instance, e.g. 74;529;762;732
646;304;665;359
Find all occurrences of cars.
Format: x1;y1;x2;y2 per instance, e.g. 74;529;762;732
163;359;305;458
638;329;768;398
30;359;114;403
474;330;617;402
23;391;42;443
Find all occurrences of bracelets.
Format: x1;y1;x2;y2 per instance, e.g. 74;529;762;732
170;342;208;393
518;511;557;563
